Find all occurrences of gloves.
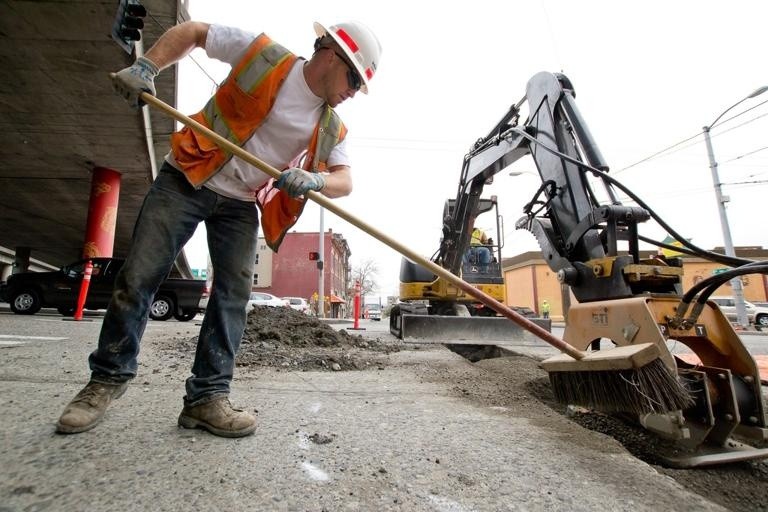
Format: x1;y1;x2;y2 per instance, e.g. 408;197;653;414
111;56;160;110
272;167;324;198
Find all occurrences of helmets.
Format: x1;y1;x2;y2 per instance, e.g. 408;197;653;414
313;14;381;95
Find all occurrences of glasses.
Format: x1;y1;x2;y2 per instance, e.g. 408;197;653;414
312;47;361;90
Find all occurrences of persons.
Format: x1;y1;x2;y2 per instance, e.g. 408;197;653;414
54;24;378;438
542;300;550;319
463;224;490;273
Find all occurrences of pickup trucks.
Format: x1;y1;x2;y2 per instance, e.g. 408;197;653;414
0;257;209;322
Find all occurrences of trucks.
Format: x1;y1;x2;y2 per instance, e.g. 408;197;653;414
363;304;383;321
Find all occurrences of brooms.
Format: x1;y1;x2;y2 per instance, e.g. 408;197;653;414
107;72;697;416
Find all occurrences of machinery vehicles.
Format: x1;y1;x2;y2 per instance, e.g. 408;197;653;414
389;70;767;469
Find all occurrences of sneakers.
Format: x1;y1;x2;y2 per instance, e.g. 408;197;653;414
56;379;128;434
178;397;258;438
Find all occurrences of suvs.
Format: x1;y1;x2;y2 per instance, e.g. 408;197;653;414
708;296;767;326
280;297;309;316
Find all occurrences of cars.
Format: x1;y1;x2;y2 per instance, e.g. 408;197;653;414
245;291;290;314
751;301;767;332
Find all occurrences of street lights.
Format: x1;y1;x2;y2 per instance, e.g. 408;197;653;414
701;84;767;329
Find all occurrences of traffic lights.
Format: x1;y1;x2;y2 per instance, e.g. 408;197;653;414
111;0;147;55
308;252;318;260
